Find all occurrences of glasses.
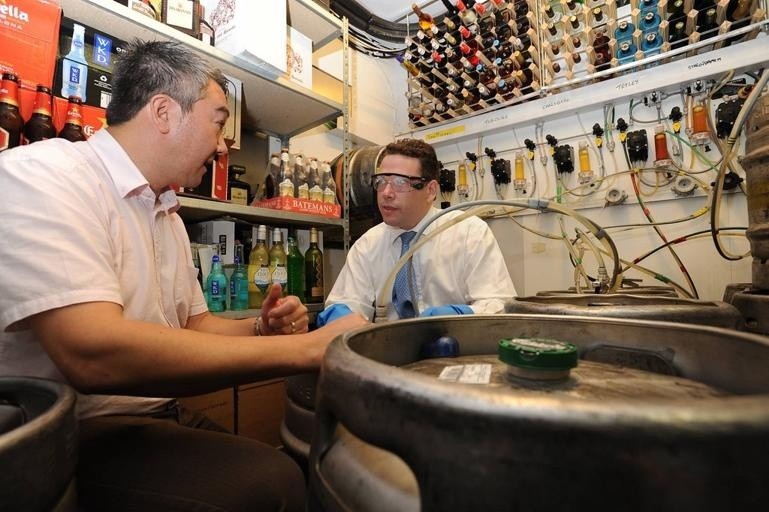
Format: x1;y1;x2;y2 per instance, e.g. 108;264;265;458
370;172;429;193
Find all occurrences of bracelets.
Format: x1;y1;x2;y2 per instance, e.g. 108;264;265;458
254;315;263;336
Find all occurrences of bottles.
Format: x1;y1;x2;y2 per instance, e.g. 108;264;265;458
24;82;59;145
456;158;466;187
205;255;227;311
227;164;251;204
403;0;768;130
515;150;525;180
654;124;669;160
0;68;23;151
578;140;592;172
58;96;86;143
61;23;89;103
265;148;337;204
231;257;249;309
247;224;323;309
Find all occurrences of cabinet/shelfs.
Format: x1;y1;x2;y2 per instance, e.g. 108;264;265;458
53;0;349;451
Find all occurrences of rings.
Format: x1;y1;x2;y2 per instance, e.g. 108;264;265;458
290;321;296;334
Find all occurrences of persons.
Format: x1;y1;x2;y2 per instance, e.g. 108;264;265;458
317;138;518;328
0;37;377;511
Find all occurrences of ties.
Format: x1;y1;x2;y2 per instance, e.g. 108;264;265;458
392;231;420;317
